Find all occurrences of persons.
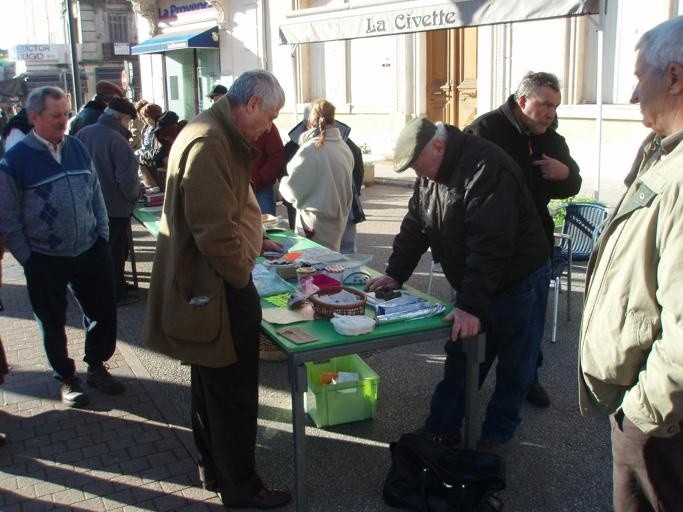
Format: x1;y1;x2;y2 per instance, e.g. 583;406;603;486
285;106;363;255
465;72;581;410
581;11;683;510
248;113;285;221
277;101;354;255
370;116;554;458
203;82;227;101
152;71;299;511
1;77;188;418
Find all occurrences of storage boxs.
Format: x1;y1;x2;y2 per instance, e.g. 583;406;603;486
301;351;379;429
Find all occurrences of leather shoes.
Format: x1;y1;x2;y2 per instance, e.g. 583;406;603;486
116;293;140;306
87;366;123;394
226;488;291;511
447;432;461;443
477;436;508;458
528;381;550;407
60;376;89;407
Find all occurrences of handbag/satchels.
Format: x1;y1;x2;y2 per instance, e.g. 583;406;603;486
382;427;507;511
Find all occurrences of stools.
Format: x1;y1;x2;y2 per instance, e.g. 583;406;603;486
426;253;456;301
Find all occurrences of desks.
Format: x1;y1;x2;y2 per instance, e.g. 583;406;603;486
130;206;480;509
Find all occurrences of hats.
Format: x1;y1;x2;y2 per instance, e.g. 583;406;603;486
206;85;227;97
153;111;179;133
393;117;436;173
109;97;138;120
97;80;123;97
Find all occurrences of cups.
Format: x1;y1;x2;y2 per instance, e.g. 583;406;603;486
298;267;314;283
324;264;344;282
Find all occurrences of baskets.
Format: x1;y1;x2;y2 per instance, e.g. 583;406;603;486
262;260;296;280
259;331;287;361
308;286;367;318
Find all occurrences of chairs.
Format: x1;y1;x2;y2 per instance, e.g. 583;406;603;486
548;202;610;267
546;233;575;341
